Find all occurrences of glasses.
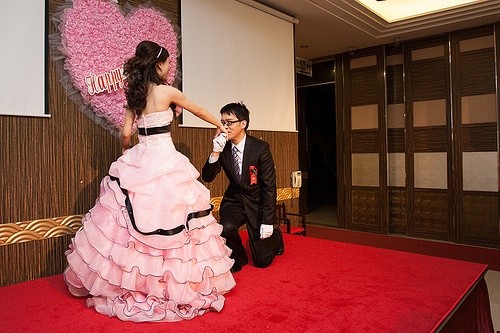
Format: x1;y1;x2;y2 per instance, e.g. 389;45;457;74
221;120;243;126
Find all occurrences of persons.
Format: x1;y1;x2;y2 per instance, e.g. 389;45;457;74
120;40;228;312
201;101;285;271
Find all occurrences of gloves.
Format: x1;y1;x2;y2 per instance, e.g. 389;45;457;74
260;224;273;239
213;133;227;152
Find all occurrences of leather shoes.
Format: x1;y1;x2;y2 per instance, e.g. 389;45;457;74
274;227;284;255
230;256;248;272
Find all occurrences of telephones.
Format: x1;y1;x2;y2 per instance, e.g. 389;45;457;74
291;171;302;187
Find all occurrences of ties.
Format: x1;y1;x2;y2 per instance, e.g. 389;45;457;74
232;146;240;175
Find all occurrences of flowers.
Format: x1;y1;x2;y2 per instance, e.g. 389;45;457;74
250;166;258;175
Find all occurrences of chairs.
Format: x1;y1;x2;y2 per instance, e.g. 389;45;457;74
277;202;306;236
